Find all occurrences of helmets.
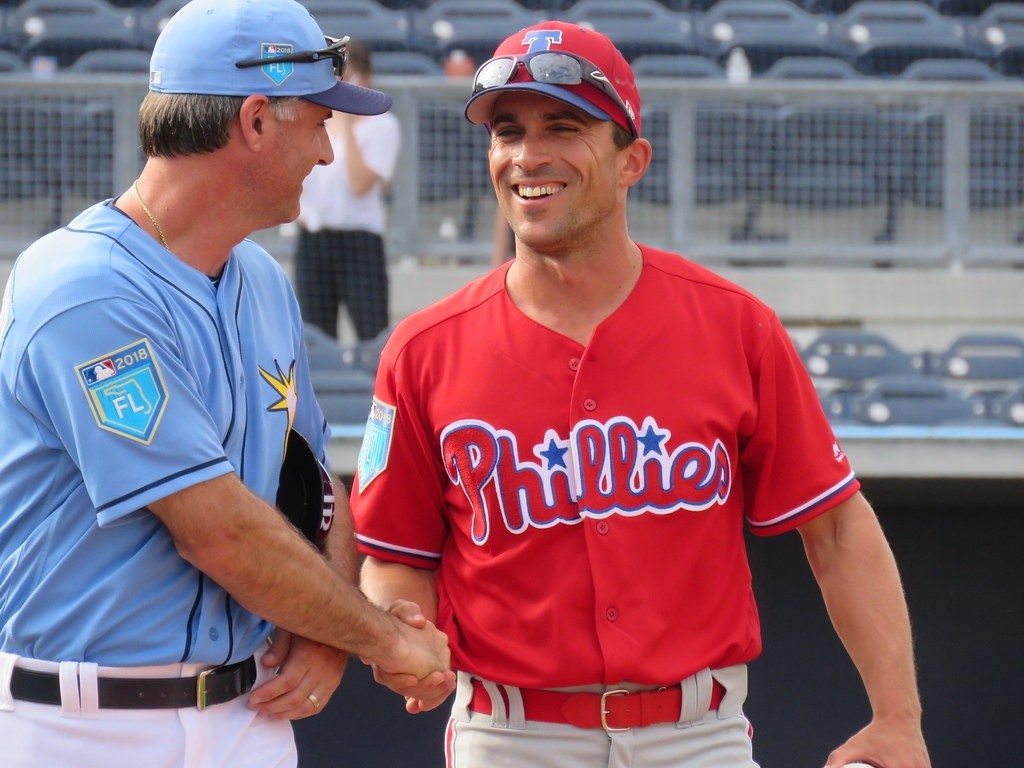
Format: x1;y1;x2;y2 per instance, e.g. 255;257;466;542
278;427;333;549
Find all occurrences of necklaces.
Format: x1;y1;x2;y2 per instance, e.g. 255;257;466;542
133;180;170;251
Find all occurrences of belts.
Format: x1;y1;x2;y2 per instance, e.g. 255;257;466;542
470;676;726;733
8;654;256;709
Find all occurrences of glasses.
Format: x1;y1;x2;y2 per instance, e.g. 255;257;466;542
237;32;352;77
471;47;641;140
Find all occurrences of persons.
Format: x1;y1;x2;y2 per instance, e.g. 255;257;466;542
351;21;933;768
292;41;402;338
0;0;452;768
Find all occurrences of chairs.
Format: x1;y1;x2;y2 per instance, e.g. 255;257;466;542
300;333;1022;439
0;0;1024;206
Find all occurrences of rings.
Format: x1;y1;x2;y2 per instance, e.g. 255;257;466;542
308;694;319;708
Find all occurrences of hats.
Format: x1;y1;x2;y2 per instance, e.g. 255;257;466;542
149;0;393;115
463;22;642;139
